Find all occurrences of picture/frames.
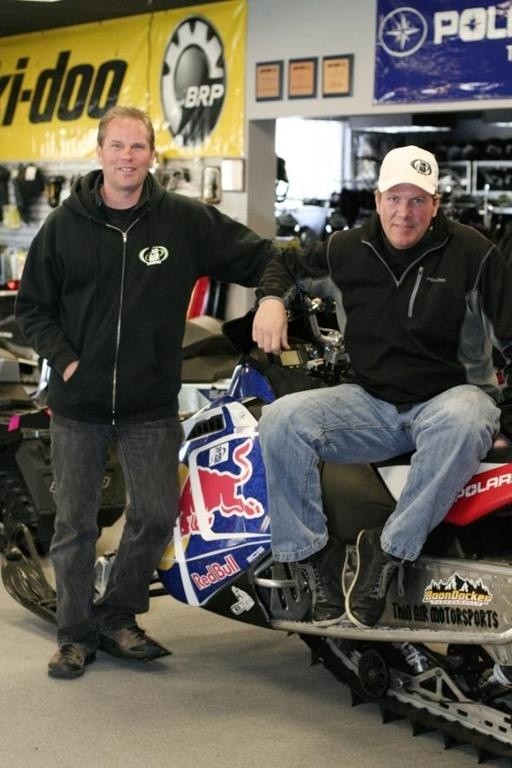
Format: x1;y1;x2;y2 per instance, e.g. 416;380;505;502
255;53;354;102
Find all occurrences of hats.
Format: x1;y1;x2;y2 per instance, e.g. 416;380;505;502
376;145;441;196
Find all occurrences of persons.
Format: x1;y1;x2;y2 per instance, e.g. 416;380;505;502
13;108;278;679
251;145;512;629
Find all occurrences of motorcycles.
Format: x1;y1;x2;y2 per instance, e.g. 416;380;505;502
0;297;512;768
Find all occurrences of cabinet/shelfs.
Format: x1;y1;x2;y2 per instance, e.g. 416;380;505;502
436;160;512;238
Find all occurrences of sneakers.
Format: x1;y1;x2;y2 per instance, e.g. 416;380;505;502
96;620;163;664
344;525;401;630
47;632;98;681
309;534;350;629
